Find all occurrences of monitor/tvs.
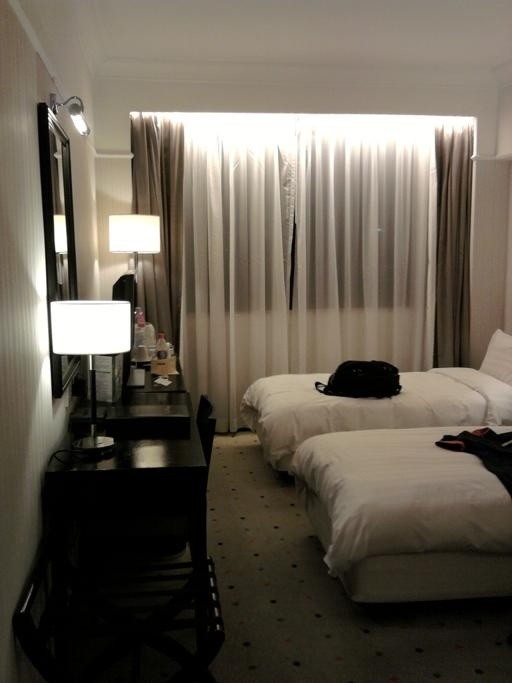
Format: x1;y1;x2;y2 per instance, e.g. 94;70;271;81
112;274;146;387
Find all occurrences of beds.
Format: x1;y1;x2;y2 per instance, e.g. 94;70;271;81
249;367;511;471
289;426;512;603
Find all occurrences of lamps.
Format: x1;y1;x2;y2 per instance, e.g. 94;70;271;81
48;298;132;456
108;212;162;310
54;214;68;299
50;91;91;136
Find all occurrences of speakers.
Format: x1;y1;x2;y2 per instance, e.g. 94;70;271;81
87;353;124;404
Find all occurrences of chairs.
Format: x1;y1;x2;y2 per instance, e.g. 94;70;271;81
82;394;219;563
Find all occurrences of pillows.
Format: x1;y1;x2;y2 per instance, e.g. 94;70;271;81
480;328;512;387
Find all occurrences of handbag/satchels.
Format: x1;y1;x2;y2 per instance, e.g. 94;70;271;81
315;359;401;398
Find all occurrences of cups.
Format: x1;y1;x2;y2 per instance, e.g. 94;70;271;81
134;345;148;361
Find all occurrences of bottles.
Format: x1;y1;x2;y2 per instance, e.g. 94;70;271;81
134;306;142;324
156;333;169;382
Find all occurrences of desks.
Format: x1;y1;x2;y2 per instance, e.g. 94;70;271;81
44;355;212;654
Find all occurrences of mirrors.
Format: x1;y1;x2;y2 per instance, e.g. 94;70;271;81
38;104;82;402
